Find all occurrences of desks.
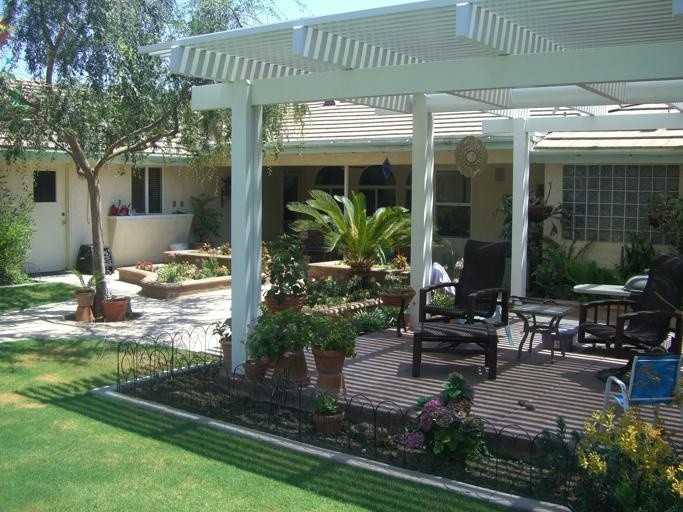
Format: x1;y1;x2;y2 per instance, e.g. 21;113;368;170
572;282;642;326
508;300;573;363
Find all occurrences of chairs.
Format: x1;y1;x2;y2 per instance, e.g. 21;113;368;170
417;238;514;345
600;351;682;429
576;252;681;381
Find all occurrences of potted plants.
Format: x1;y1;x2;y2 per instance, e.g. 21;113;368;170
181;193;223;249
68;268;133;324
214;235;360;433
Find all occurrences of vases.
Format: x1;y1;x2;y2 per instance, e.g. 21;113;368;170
378;288;416;305
422;446;469;479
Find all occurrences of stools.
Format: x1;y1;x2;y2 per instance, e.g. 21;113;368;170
410;322;501;382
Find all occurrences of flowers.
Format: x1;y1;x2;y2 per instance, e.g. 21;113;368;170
536;408;682;512
396;370;494;462
376;254;414;292
